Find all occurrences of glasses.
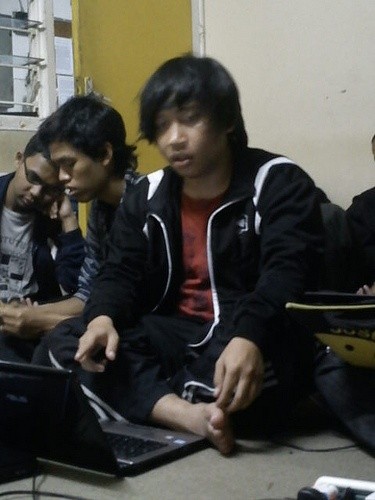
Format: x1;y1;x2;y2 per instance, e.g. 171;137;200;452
23;156;62;195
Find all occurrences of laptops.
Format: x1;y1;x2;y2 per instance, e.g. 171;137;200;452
1;361;212;481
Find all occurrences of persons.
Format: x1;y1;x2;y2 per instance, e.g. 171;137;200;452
316;132;374;451
0;96;141;372
0;133;86;361
48;55;318;457
